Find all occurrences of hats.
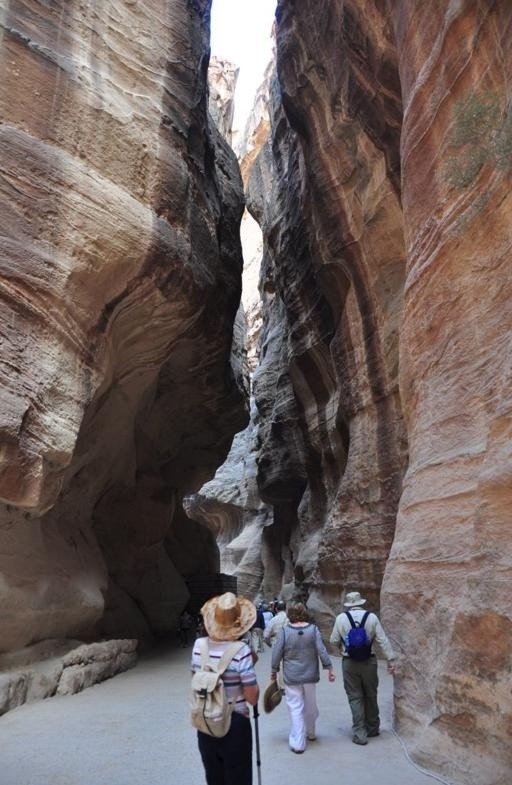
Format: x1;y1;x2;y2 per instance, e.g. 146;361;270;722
264;680;285;713
200;592;257;640
344;591;367;607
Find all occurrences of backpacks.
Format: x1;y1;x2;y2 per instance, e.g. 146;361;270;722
189;637;246;738
341;611;374;662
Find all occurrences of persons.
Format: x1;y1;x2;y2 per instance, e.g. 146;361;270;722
190;592;260;785
270;602;335;754
240;596;289;656
176;610;205;648
329;590;396;745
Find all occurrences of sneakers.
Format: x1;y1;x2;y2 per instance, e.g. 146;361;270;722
291;747;304;754
308;734;317;740
352;728;380;745
